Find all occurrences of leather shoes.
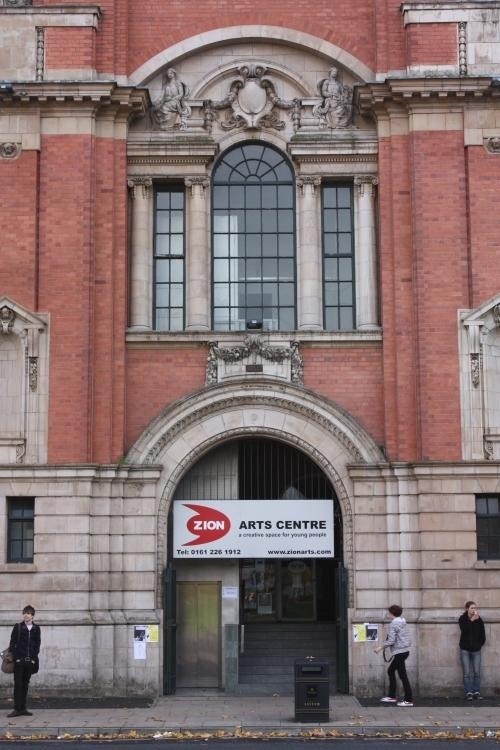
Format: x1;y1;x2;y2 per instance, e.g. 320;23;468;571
7;709;33;718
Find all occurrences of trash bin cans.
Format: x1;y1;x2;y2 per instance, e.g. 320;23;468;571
294;657;329;723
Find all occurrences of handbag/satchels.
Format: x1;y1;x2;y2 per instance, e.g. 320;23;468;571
32;657;40;674
0;647;16;673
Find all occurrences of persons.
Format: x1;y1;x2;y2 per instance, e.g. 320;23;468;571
373;605;415;707
459;602;486;700
323;67;346;126
6;605;41;716
153;69;184;130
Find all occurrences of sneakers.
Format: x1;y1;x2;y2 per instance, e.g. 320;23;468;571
465;691;484;701
379;695;414;707
